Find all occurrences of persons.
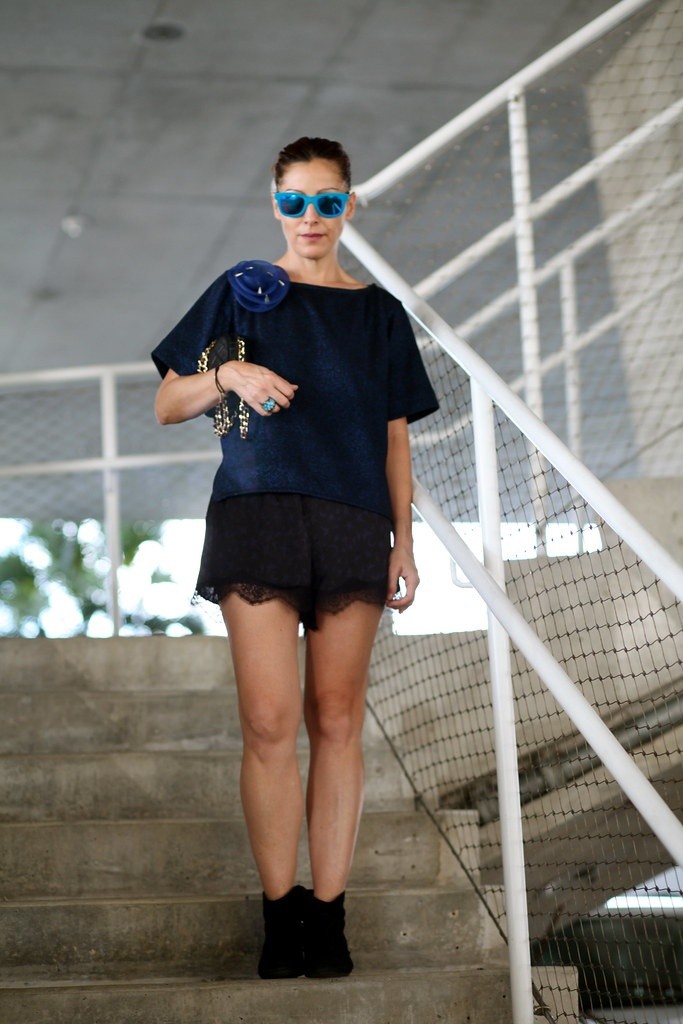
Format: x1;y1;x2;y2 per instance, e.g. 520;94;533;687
151;137;439;978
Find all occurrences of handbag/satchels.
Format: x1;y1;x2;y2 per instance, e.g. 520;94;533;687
198;333;249;440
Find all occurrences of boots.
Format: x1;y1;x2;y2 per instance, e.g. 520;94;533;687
258;886;303;978
305;888;353;977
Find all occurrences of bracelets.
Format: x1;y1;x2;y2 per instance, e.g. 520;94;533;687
215;365;225;394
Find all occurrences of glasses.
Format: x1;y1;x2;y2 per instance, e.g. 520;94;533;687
274;192;349;218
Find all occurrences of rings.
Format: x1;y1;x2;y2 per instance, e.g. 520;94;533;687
262;398;276;412
